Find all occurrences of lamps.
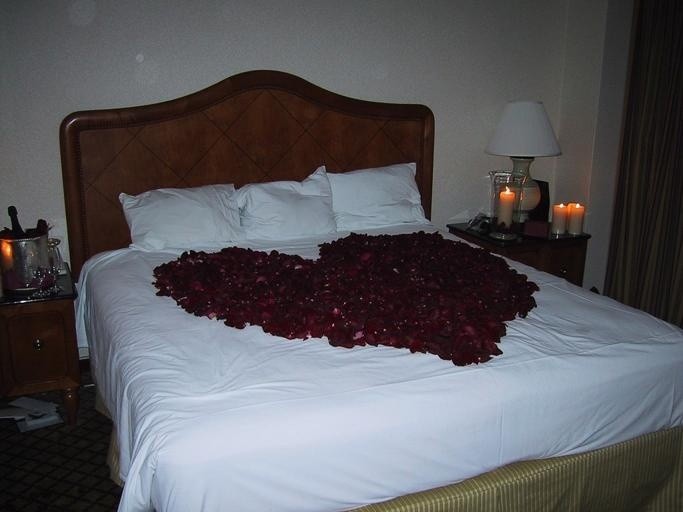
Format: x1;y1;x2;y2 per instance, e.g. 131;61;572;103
483;100;564;223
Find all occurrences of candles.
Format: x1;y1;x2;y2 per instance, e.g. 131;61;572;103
497;191;515;229
549;202;587;236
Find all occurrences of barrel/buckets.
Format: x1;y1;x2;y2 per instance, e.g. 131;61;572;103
0;228;53;291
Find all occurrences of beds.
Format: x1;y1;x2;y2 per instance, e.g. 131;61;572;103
58;67;683;512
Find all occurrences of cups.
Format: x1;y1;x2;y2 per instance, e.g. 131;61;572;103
550;203;586;239
485;169;526;242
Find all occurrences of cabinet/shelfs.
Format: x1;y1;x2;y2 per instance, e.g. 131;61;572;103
446;220;593;289
0;262;80;419
